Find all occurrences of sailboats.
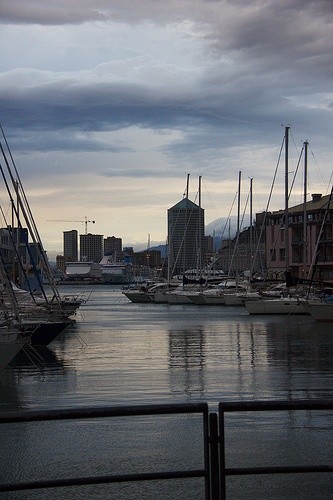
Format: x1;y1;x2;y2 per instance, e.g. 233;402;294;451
120;124;333;321
1;122;84;346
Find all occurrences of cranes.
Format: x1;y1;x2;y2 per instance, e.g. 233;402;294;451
47;216;95;235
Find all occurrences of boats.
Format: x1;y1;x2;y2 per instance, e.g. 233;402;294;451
99;263;131;284
64;258;101;282
